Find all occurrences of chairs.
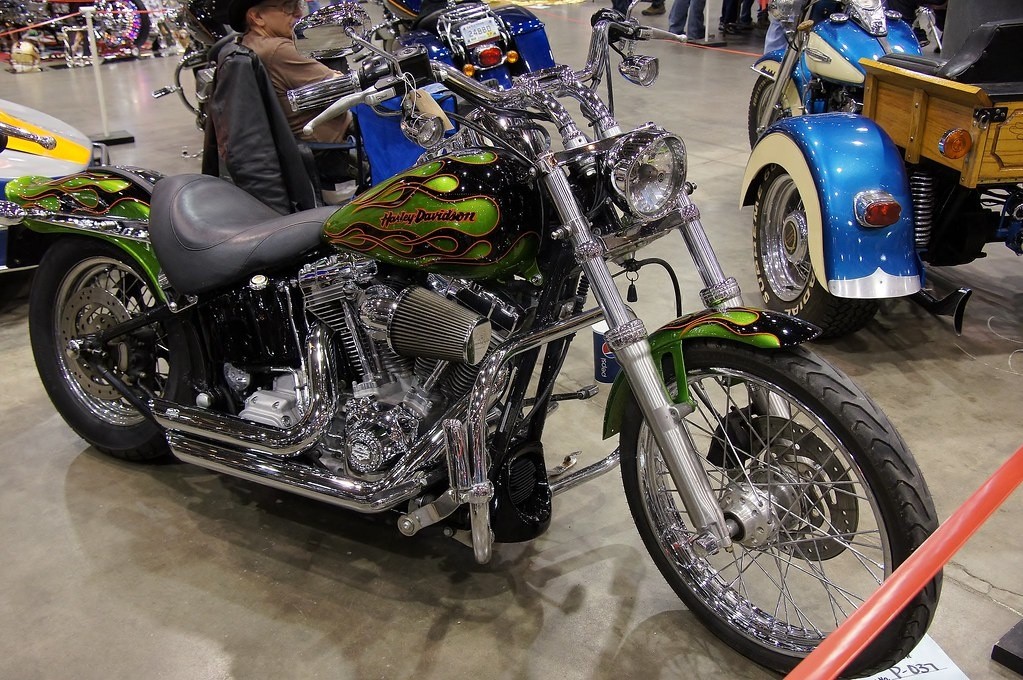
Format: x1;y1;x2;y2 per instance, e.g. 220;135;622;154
204;43;365;214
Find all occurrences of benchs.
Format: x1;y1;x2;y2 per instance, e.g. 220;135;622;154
859;1;1023;190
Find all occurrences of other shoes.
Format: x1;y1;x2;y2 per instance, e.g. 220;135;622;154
719;20;756;30
689;33;714;40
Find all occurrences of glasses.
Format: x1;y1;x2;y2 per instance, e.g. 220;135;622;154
261;0;303;14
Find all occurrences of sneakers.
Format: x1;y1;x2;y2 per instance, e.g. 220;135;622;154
642;4;666;15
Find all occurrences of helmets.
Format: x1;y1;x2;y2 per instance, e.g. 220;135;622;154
11;40;40;67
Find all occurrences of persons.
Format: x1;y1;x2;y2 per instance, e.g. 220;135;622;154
669;0;706;40
642;0;666;16
764;0;802;53
611;0;633;16
887;0;948;53
215;0;370;183
718;0;771;34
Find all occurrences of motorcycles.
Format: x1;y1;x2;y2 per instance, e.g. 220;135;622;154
0;0;563;323
738;0;1023;347
1;0;947;680
